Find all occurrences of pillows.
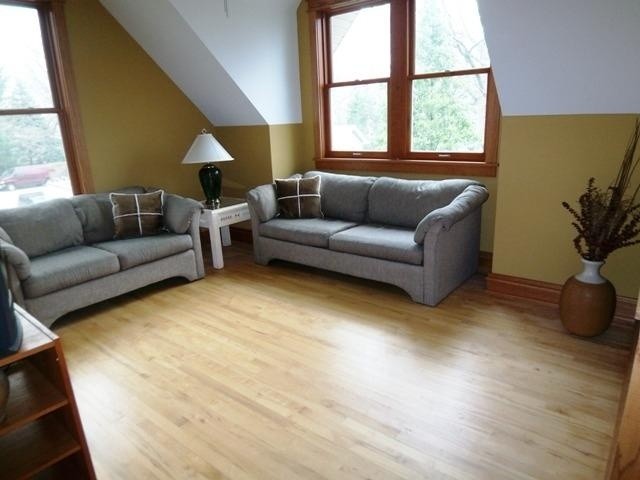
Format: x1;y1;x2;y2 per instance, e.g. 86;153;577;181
107;188;167;239
272;174;324;219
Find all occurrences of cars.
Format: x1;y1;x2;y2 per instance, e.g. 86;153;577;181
0;161;60;191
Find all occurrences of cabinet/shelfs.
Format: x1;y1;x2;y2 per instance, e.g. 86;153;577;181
1;298;100;479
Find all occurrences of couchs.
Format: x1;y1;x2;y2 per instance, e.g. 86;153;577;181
243;168;491;310
0;185;205;331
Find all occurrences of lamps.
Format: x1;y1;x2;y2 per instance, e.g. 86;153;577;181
177;128;237;209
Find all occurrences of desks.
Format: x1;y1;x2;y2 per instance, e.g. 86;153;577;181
197;194;252;270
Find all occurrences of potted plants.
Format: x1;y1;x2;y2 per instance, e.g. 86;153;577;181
556;114;640;343
